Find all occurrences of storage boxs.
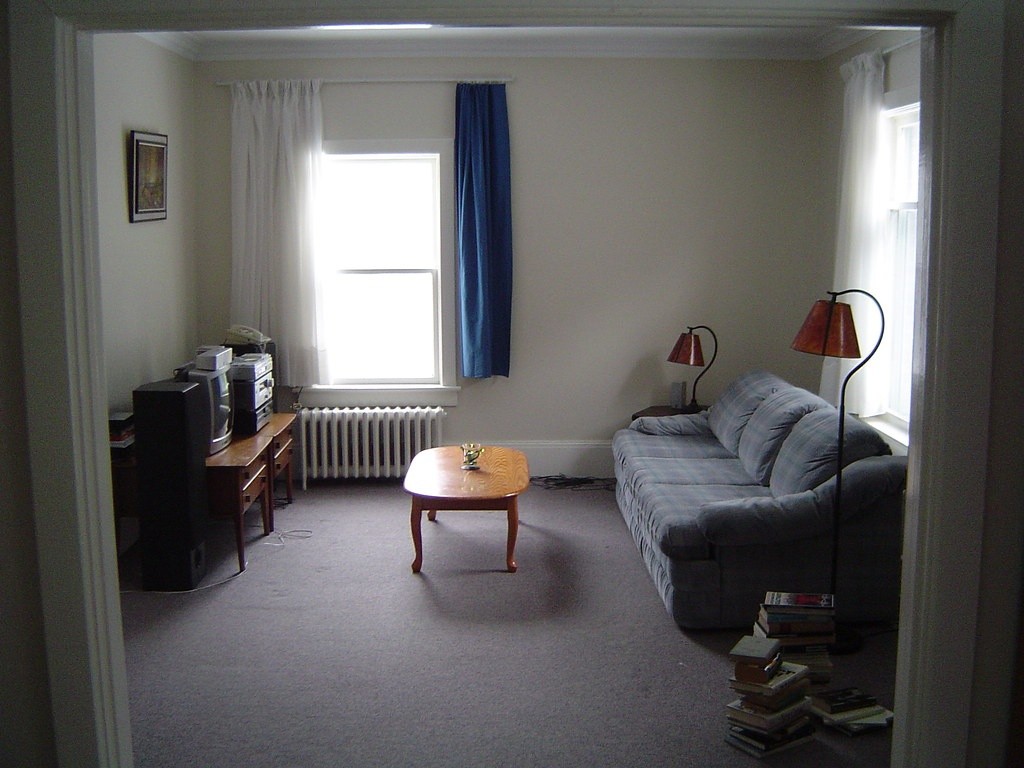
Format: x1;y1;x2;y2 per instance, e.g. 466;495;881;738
671;381;686;409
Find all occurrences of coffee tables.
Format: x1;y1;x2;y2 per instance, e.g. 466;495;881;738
403;445;530;574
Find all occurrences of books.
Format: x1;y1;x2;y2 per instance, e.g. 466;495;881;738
721;593;893;759
729;637;783;663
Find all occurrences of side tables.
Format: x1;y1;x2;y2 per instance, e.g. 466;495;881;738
632;406;712;421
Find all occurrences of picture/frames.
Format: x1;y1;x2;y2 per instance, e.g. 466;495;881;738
129;130;168;223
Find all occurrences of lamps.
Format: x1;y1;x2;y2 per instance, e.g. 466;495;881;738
667;326;718;414
790;289;885;658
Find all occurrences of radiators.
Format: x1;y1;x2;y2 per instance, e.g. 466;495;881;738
297;407;448;490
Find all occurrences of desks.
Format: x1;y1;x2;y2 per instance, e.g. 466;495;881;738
113;412;298;572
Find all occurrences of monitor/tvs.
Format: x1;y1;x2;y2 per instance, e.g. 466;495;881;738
168;364;235;455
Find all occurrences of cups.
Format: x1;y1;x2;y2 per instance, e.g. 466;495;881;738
461;443;484;470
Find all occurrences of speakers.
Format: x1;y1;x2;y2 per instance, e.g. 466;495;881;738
133;382;206;591
220;342;278;413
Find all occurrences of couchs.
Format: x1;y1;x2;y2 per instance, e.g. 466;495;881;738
612;370;908;630
